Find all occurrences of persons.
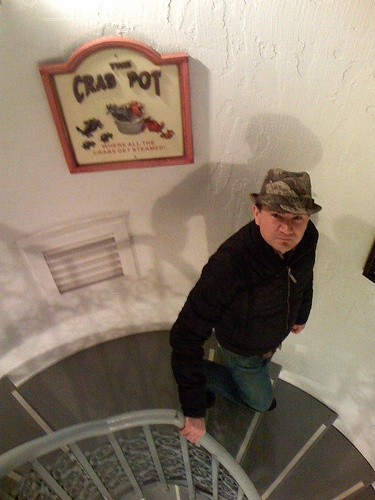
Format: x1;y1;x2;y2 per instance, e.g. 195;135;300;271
169;168;322;444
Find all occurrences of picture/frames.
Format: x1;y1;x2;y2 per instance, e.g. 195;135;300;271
39;38;194;174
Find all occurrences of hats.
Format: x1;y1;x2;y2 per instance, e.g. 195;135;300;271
248;168;322;215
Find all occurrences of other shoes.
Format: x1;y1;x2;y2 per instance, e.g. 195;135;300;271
206;390;215;408
266;398;277;412
